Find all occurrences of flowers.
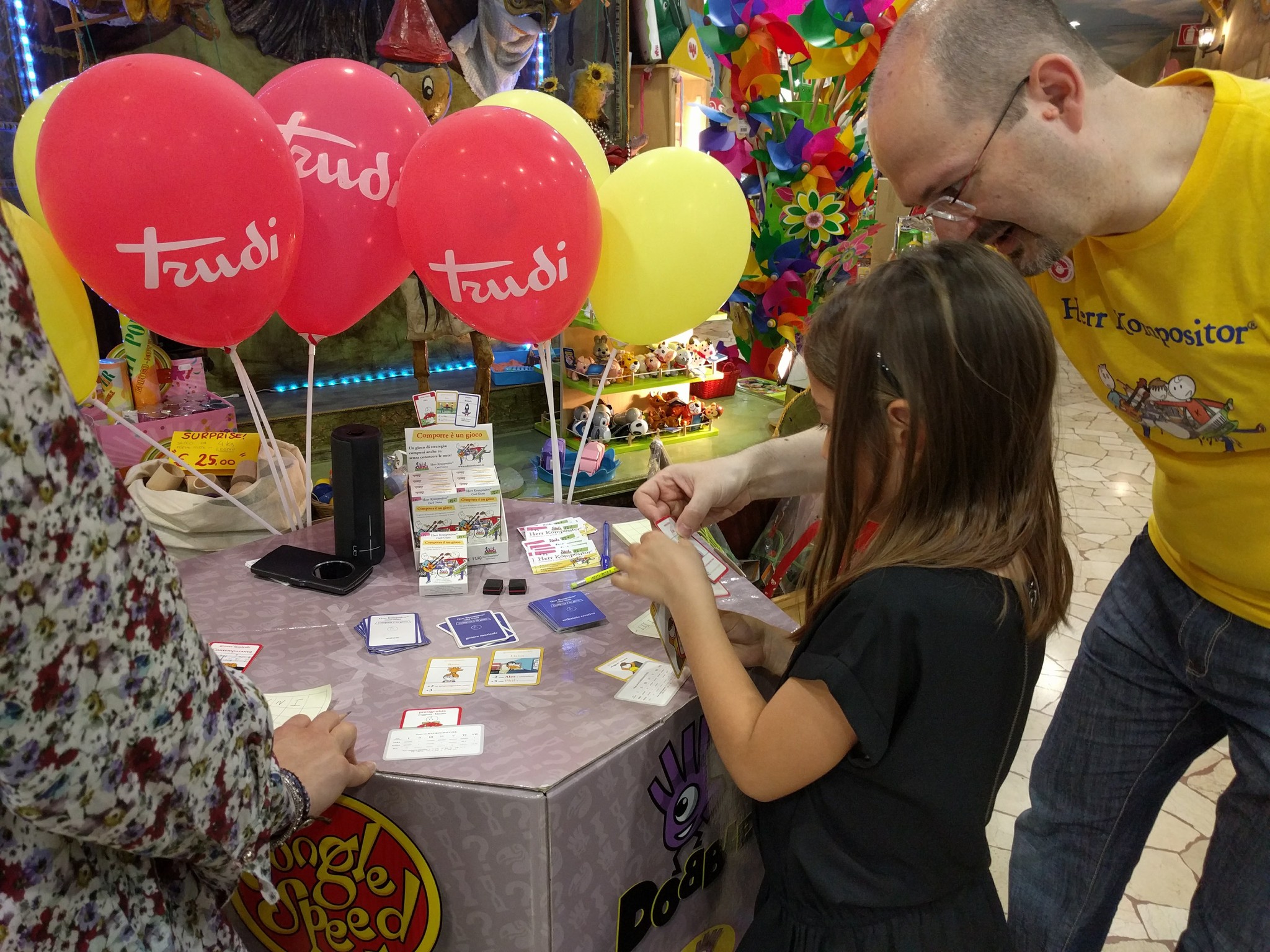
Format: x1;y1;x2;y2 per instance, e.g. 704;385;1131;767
728;301;752;341
538;62;615;124
774;186;874;281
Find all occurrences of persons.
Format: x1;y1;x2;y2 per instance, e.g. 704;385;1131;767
612;243;1073;952
633;1;1270;952
0;203;374;952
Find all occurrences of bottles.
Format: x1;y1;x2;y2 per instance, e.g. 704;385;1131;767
384;464;408;501
330;423;385;565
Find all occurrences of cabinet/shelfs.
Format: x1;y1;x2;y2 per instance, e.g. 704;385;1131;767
534;298;724;455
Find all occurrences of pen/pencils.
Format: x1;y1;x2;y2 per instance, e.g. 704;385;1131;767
570;566;619;589
601;521;610;568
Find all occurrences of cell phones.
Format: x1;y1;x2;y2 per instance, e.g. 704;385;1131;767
250;545;374;596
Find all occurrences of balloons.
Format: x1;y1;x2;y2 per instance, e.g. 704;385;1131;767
4;50;752;405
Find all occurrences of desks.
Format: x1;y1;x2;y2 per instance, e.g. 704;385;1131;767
305;386;783;505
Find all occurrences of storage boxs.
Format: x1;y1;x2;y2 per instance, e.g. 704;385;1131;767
169;490;765;952
85;391;238;510
509;579;527;595
490;348;561;386
419;531;469;596
408;467;502;547
483;579;503;595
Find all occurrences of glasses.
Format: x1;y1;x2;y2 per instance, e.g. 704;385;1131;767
898;76;1036;235
